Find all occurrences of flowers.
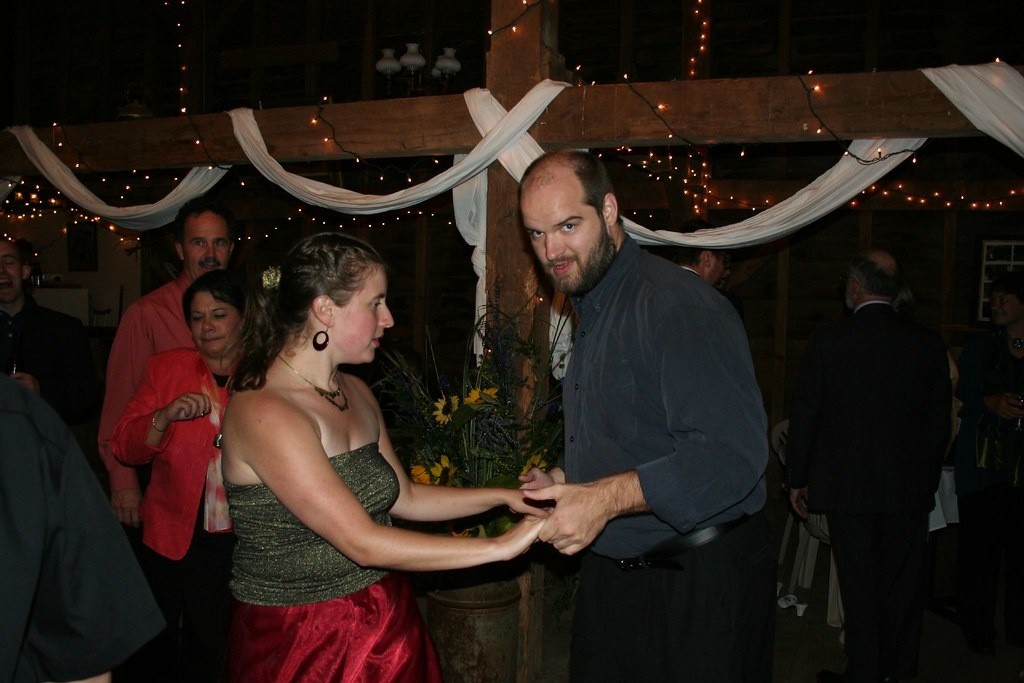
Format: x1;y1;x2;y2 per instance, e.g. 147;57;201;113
368;268;574;542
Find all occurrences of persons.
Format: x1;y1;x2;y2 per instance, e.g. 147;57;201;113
0;149;1024;683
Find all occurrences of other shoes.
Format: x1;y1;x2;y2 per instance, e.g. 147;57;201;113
816;669;839;683
965;630;996;654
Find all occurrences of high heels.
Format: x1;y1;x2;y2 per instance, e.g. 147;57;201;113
777;596;807;616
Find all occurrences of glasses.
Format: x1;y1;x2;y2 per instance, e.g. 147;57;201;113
716;253;732;268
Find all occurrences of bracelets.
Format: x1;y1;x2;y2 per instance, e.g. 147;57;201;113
152;409;168;434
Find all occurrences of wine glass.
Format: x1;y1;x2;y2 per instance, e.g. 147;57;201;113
1011;392;1024;431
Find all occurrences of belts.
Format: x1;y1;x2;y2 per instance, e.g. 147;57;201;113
606;513;749;572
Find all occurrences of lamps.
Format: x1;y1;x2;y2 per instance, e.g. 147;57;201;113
375;40;467;96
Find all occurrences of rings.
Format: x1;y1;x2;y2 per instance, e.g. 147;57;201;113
186;395;191;404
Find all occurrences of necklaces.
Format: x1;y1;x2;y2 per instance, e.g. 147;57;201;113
276;354;349;412
